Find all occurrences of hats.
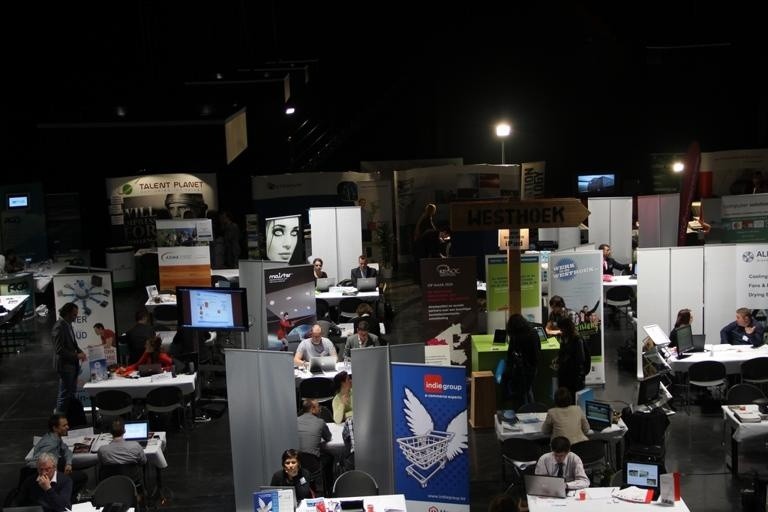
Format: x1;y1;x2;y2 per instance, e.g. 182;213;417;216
166;193;208;208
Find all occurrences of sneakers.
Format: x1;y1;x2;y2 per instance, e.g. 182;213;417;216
194;414;212;424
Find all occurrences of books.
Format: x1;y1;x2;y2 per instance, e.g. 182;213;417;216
733;408;761;423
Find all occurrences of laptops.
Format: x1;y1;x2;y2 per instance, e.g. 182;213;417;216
316;278;334;293
139;364;162;377
525;474;569;499
585;400;611;432
307;356;336;371
683;334;705;352
123;421;148;449
620;461;660;501
357;277;376;292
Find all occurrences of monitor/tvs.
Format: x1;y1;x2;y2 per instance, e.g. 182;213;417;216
637;374;661;411
675;324;694;360
176;285;249;332
175;352;198;375
261;485;298;512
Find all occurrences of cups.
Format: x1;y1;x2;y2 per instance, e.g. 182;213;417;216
367;504;373;512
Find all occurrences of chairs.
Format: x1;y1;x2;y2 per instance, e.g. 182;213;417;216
0;251;410;512
469;329;768;512
602;285;636;319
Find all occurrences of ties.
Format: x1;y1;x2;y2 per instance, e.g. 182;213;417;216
557;463;564;478
603;258;607;271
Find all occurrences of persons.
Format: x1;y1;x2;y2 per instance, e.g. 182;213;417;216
278;312;295;350
182;210;240;269
669;309;712;397
411;203;458;277
351;254;377;287
599;243;633;277
313;257;329;319
164;193;206;220
719;307;765;345
293;304;380;477
269;448;310;506
266;217;300;262
500;295;669;490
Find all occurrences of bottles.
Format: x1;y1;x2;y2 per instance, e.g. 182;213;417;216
579;490;586;499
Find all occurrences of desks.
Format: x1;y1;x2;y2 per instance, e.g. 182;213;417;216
600;270;640;292
